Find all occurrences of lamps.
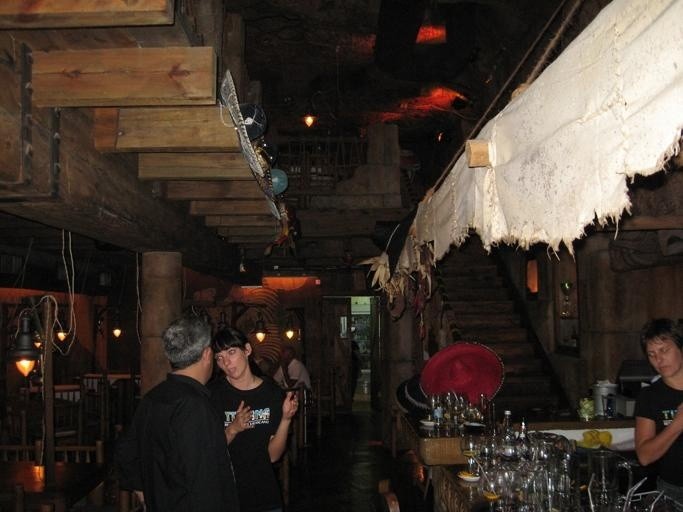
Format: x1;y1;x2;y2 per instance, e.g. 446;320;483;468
55;308;71;343
284;307;305;339
234;301;269;343
9;305;41;379
91;302;123;351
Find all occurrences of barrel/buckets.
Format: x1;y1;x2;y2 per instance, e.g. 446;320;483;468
590;382;619;417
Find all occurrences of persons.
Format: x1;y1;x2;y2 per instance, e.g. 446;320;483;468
118;312;299;512
633;317;683;512
273;343;312;398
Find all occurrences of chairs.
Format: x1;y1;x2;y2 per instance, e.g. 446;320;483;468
1;371;142;512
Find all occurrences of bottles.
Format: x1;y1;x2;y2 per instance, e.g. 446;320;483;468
518;414;531;462
587;451;619;511
428;393;497;431
500;409;517;462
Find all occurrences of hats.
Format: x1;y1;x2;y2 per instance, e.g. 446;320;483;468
395;342;505;417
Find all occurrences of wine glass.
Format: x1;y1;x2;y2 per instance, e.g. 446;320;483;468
456;431;578;512
